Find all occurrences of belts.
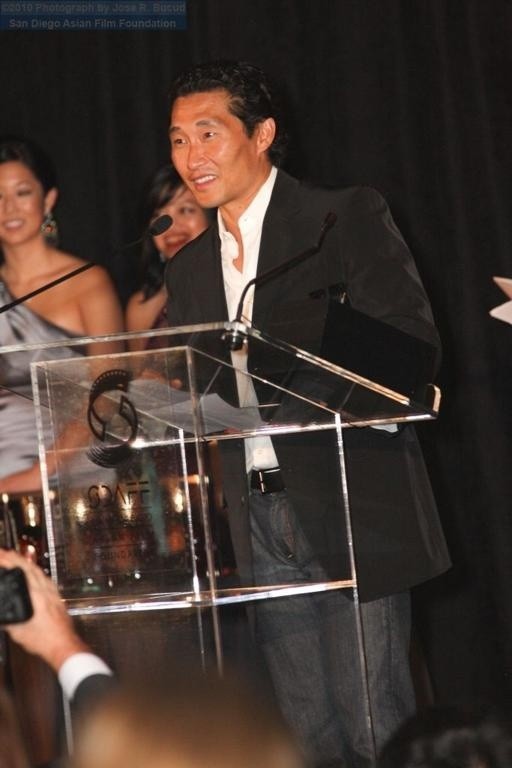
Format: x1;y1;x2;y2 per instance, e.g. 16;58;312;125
251;468;288;494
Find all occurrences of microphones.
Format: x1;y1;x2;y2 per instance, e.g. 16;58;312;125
0;215;173;313
225;211;336;351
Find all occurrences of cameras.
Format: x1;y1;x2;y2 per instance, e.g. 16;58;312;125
0;566;32;626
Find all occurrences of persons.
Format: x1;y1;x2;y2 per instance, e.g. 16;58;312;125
131;54;459;768
66;663;310;766
118;156;210;359
1;136;129;507
0;544;119;767
376;700;512;767
